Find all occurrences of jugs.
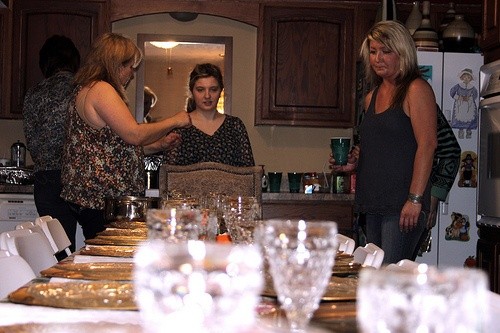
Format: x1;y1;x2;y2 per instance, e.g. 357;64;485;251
11;139;25;168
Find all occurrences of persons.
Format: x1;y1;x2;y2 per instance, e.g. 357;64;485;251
329;20;460;264
24;33;193;261
163;63;256;169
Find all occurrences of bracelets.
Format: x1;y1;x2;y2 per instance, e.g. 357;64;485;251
406;192;422;204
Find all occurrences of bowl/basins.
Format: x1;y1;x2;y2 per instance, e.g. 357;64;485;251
0;165;34;187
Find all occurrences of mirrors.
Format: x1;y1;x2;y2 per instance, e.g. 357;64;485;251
134;33;233;125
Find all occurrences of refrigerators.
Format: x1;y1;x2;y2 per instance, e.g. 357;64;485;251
411;50;482;272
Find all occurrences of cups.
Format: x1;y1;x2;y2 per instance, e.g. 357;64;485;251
261;136;356;194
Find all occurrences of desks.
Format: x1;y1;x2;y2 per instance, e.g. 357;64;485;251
0;226;500;333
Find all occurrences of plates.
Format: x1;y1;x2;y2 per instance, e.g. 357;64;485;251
0;221;359;333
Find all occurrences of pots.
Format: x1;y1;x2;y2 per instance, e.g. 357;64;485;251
106;195;155;224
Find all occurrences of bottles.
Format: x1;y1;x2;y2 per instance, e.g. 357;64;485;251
404;0;474;53
0;152;10;167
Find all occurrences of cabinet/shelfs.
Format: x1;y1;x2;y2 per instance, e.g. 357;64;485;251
261;201;353;237
0;0;101;120
475;237;500;295
254;6;355;127
482;0;500;53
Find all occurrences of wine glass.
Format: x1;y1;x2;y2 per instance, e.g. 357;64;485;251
128;189;486;333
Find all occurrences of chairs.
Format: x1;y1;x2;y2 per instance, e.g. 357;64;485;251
0;215;72;301
158;162;263;221
